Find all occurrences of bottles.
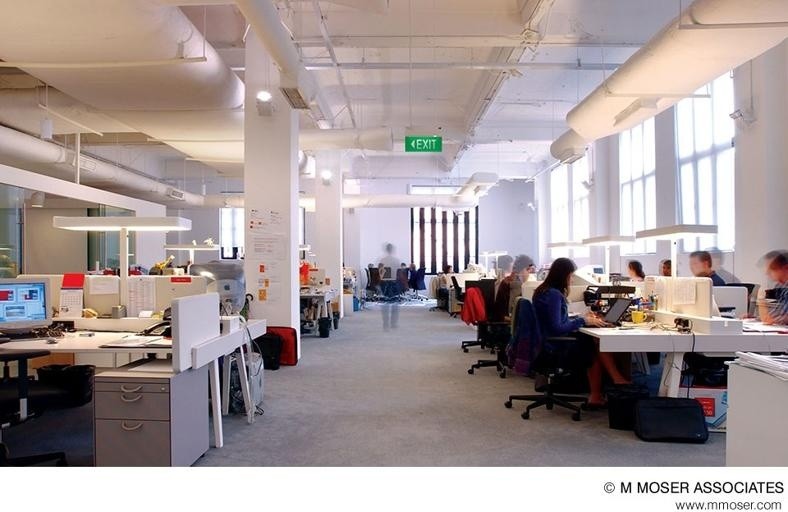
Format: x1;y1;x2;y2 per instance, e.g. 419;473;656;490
240;246;243;256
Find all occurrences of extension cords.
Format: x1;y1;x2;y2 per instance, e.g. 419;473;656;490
673;318;692;331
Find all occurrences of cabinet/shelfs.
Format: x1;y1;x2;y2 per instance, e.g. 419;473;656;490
92;358;210;467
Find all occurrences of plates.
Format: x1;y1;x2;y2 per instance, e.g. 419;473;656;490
88;271;104;275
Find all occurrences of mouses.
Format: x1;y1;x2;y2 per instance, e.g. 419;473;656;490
48;338;59;344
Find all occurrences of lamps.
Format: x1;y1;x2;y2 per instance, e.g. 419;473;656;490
478;249;507;268
36;105;56;142
255;91;272;117
162;243;219;263
321;168;332;186
547;224;719;275
52;216;192;312
31;192;45;208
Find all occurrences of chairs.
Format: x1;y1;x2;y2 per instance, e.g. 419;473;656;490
0;348;70;465
427;276;787;430
365;265;429;301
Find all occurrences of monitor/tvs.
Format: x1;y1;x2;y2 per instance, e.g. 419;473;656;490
0;277;51;335
593;267;603;273
713;286;748;319
190;264;246;314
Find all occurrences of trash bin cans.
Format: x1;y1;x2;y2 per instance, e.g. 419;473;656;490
62;364;96;404
328;311;340;329
318;316;332;338
605;384;649;431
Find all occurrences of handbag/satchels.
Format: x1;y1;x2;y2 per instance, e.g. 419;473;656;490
634;396;708;443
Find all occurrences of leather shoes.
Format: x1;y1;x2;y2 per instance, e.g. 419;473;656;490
584;397;608;409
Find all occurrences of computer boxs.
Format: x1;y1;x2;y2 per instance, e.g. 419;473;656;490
229;353;263;417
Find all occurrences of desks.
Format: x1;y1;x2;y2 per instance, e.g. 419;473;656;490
0;329;226;450
221;314;268;425
298;243;360;335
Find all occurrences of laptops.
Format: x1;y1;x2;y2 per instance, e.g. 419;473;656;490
583;297;632;329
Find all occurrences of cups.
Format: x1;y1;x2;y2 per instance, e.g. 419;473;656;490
111;305;127;319
221;247;224;257
631;310;647;323
95;261;100;271
232;246;238;259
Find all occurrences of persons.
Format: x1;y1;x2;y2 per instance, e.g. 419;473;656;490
368;263;417;297
532;257;635;411
658;258;679;278
684;246;741;376
377;243;403;329
492;254;513;281
627;260;646;282
486;254;537;365
711;249;788;386
442;265;453;274
